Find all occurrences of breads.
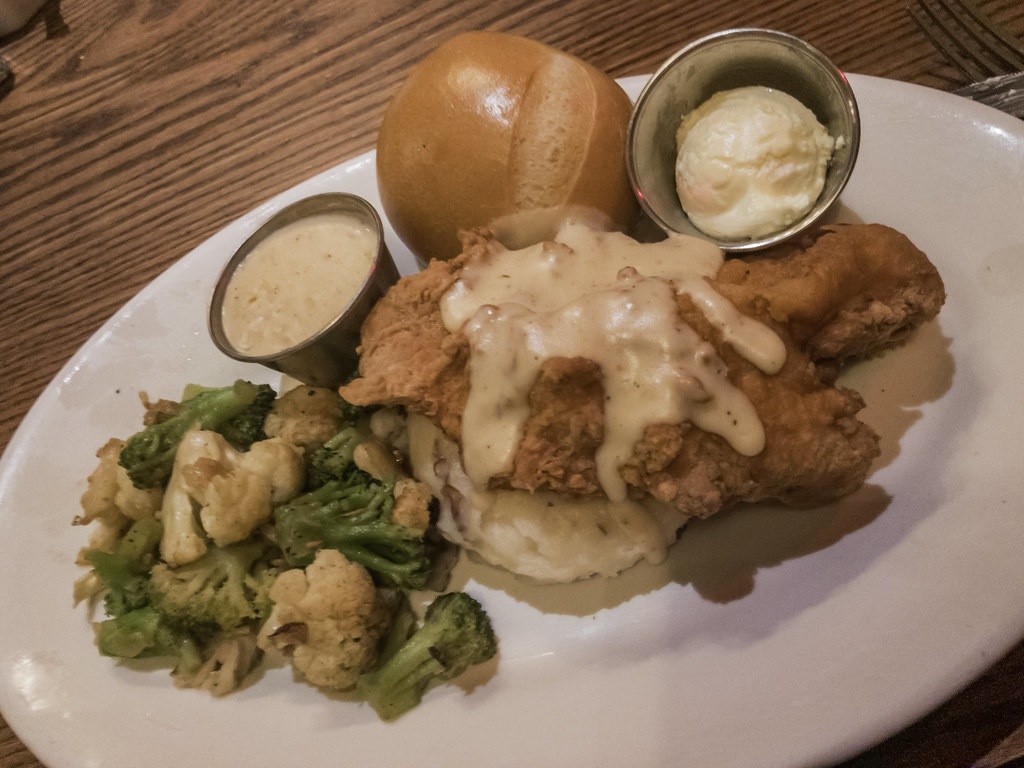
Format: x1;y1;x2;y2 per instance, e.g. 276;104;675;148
375;28;635;264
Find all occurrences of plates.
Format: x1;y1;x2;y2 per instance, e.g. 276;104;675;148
0;74;1024;768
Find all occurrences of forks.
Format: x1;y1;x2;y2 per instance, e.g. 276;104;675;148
910;15;1024;82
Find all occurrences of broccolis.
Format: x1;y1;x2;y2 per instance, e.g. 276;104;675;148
68;380;496;724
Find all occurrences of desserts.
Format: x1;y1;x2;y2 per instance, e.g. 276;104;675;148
674;85;836;243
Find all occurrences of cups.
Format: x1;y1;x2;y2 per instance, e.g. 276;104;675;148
626;28;861;254
207;191;401;390
